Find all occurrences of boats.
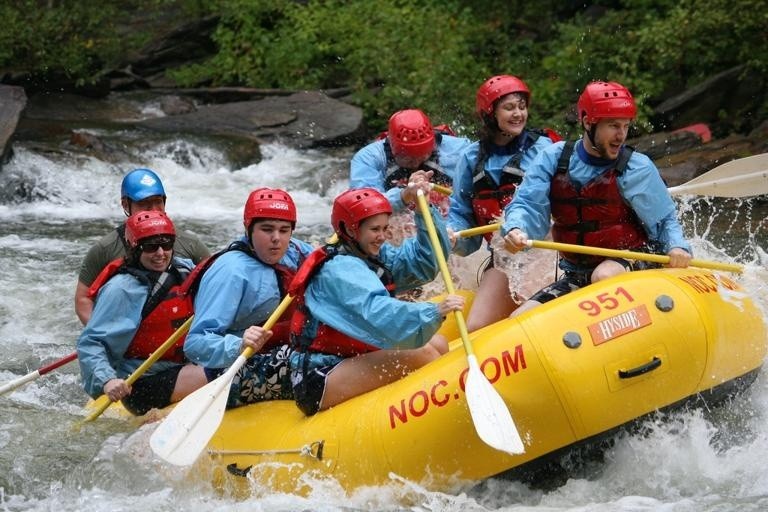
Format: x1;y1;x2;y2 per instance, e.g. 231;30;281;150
82;259;767;506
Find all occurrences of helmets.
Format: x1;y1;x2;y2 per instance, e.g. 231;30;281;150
243;188;296;230
125;211;176;252
388;108;435;161
577;81;637;130
476;75;531;125
121;169;166;202
331;188;393;243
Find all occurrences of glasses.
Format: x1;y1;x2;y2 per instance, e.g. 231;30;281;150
138;239;175;253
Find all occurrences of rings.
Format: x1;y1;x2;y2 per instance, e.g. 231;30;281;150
119;389;124;395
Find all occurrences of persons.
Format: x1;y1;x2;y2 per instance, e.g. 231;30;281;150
349;110;474;303
445;76;564;335
501;81;693;318
77;210;210;415
287;167;465;417
176;187;317;413
77;168;209;326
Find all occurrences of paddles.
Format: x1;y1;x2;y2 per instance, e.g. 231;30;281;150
149;292;295;465
409;182;525;454
